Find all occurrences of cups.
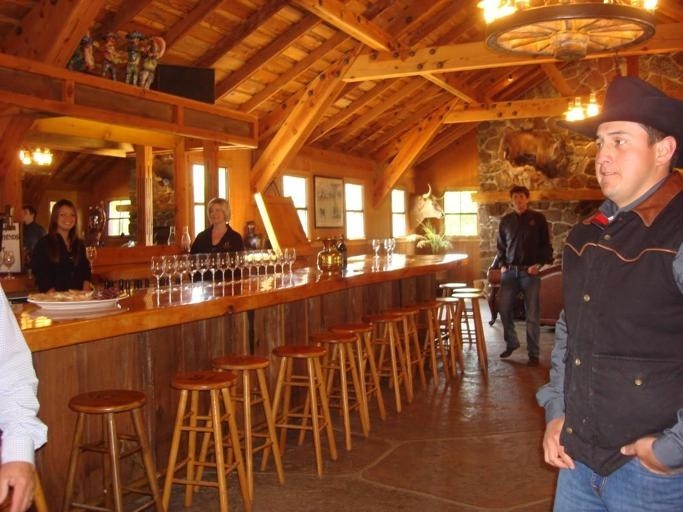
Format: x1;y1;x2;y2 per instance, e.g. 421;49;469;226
369;237;394;260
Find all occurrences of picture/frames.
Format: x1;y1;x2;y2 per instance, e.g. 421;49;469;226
312;174;344;229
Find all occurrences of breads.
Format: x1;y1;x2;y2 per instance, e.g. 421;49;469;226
29;288;92;302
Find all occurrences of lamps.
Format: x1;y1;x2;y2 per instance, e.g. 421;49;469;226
472;1;662;59
564;92;602;123
21;149;57;177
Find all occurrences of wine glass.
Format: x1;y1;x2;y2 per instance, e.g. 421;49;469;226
150;247;296;301
2;251;14;280
85;245;96;272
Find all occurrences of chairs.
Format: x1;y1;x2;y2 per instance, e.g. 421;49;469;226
532;264;563;325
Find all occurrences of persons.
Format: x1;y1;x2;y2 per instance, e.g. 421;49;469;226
0;270;49;510
22;202;44;249
533;75;682;512
29;196;96;297
491;183;554;364
184;194;248;283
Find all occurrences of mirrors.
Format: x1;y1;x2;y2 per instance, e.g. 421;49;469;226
21;148;176;254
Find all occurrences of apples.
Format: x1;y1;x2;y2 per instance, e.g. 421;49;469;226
527;266;537;274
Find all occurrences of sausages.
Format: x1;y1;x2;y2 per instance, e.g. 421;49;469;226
102;287;116;299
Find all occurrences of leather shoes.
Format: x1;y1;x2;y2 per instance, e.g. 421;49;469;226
527;357;539;367
500;343;521;358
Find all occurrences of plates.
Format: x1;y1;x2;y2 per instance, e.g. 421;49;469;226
25;292;130;311
29;303;129;322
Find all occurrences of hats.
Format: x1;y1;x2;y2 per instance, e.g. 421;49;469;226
555;76;682;143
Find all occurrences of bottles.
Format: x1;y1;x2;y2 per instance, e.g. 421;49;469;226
181;227;190;251
103;279;149;290
167;226;175;246
337;235;347;269
